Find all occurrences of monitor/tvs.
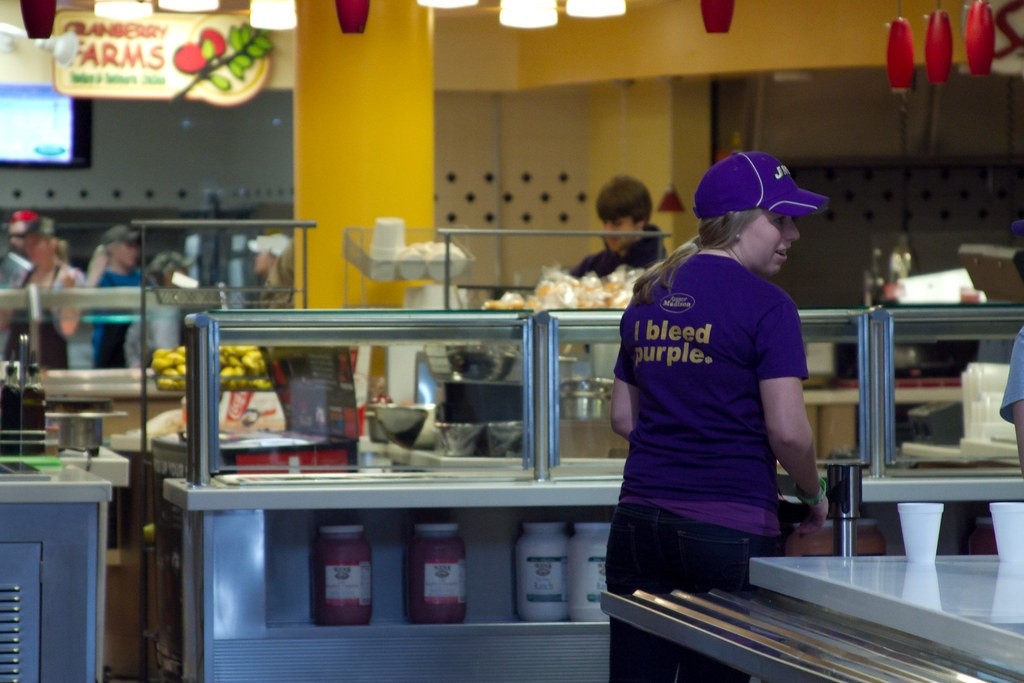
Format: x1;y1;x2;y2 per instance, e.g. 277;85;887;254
0;84;93;169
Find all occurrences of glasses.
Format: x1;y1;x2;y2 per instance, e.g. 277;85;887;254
9;228;34;238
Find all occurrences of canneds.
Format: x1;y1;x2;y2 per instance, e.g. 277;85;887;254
310;522;612;624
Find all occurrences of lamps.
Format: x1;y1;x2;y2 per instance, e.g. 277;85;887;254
966;0;998;80
925;0;953;87
887;0;914;94
17;0;743;41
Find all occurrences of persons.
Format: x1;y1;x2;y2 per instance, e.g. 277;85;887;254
124;251;194;369
0;211;94;370
569;176;666;277
87;224;149;368
247;232;295;308
998;220;1024;481
604;150;829;683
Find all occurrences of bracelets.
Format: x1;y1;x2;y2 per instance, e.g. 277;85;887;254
794;479;826;505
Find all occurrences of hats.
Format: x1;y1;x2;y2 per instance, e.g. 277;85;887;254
102;225;140;245
248;233;290;256
144;251;195;279
693;151;829;217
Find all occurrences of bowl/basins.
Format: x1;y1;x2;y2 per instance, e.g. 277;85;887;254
443;345;516;381
556;374;614;420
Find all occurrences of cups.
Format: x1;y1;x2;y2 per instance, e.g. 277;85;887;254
437;422;487;456
366;403;427;446
992;568;1024;625
990;502;1022;567
370;217;472;312
487;420;525;458
897;502;943;563
902;563;941;612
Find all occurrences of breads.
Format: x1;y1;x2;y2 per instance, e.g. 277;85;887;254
481;272;637;309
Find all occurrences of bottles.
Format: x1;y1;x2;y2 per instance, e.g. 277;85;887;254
407;523;465;623
0;351;19;455
568;522;615;619
22;352;45;455
862;236;913;308
516;522;567;622
315;524;374;624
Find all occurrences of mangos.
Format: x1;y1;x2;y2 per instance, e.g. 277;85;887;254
150;341;274;392
